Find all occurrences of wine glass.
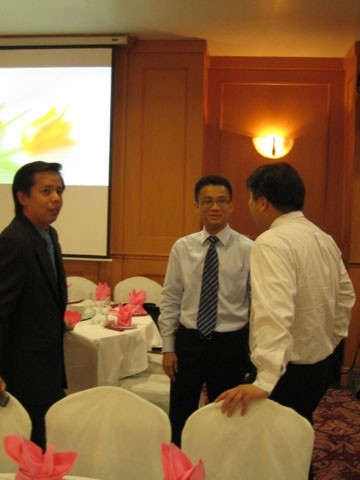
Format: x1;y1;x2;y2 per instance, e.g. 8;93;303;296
103;309;110;325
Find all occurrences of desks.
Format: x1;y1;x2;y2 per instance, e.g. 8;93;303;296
64;300;163;387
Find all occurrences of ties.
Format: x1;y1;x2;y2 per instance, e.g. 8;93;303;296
196;235;220;337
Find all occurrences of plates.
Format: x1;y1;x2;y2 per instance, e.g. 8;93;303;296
111;324;137;329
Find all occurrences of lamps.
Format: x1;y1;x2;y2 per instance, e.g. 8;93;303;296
253;136;295;159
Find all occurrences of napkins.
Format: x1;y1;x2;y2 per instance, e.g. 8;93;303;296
64;310;81;327
96;281;111;300
4;435;79;480
125;288;148;314
161;439;206;480
109;301;132;327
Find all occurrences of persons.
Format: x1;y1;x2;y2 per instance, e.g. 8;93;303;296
157;175;257;449
0;161;68;453
215;163;356;418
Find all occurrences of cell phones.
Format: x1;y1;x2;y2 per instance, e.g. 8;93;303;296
0;393;10;407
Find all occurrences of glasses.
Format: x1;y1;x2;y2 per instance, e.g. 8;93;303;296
199;199;230;206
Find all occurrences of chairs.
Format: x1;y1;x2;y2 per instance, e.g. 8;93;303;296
1;277;316;480
